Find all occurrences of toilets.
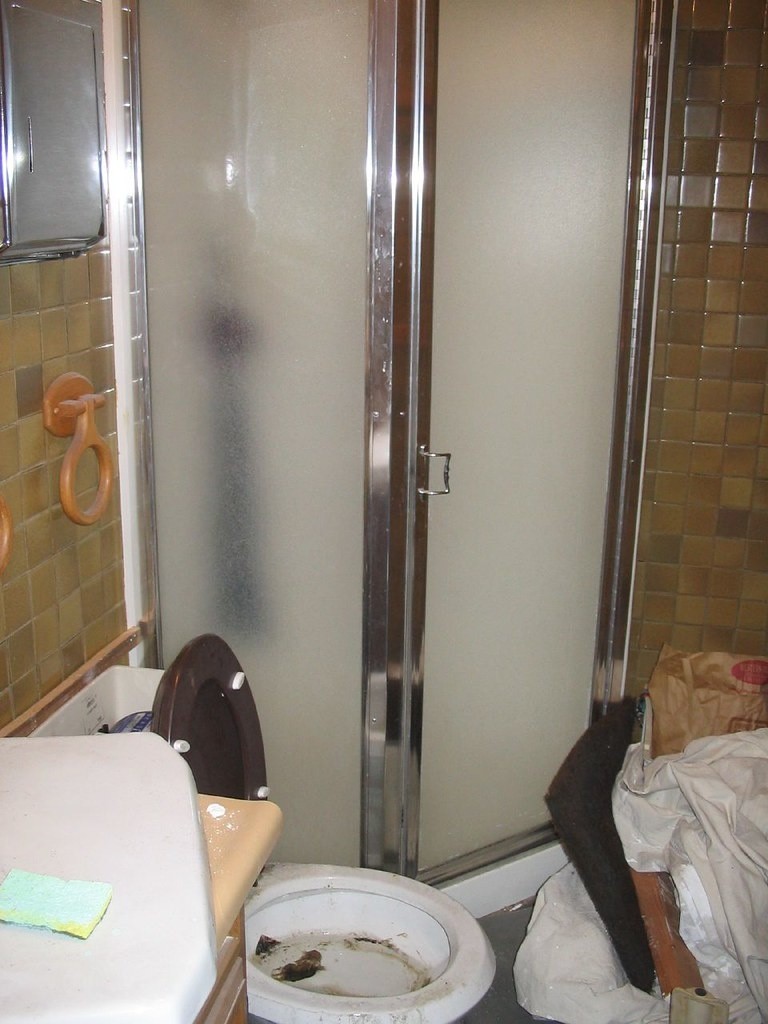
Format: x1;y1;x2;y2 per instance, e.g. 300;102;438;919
28;633;496;1024
